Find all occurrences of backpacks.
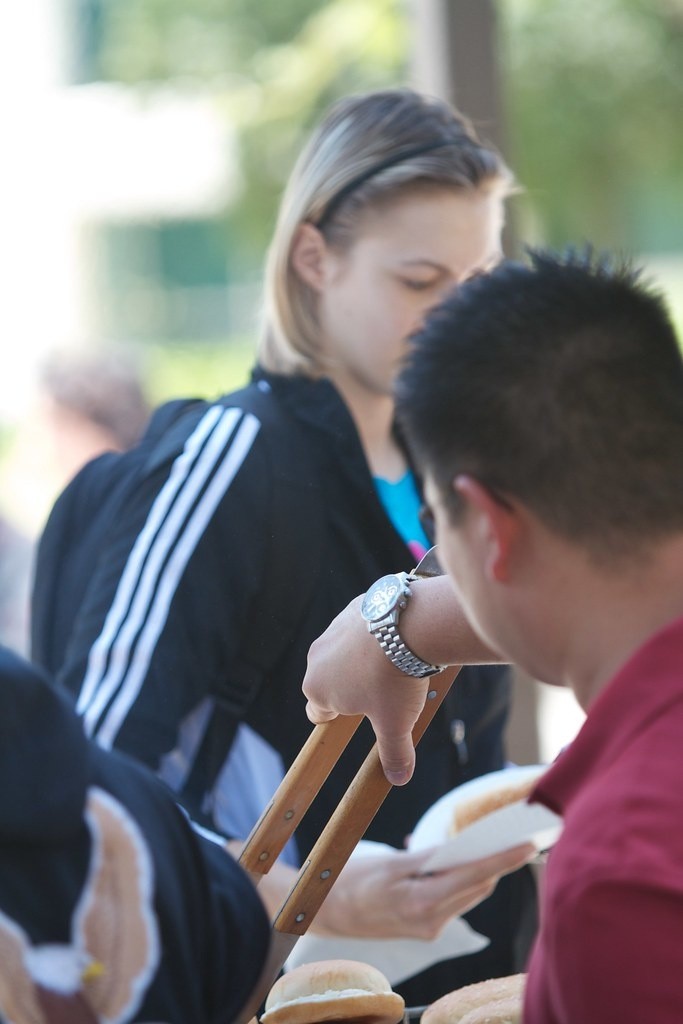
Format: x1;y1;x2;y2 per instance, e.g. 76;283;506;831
31;388;286;821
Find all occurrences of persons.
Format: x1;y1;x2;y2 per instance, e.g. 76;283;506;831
301;242;683;1024
0;642;274;1024
55;87;542;1006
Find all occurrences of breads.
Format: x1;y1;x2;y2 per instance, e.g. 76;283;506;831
257;959;405;1024
403;762;551;854
417;970;527;1024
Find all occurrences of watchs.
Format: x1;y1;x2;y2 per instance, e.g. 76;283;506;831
360;571;448;679
